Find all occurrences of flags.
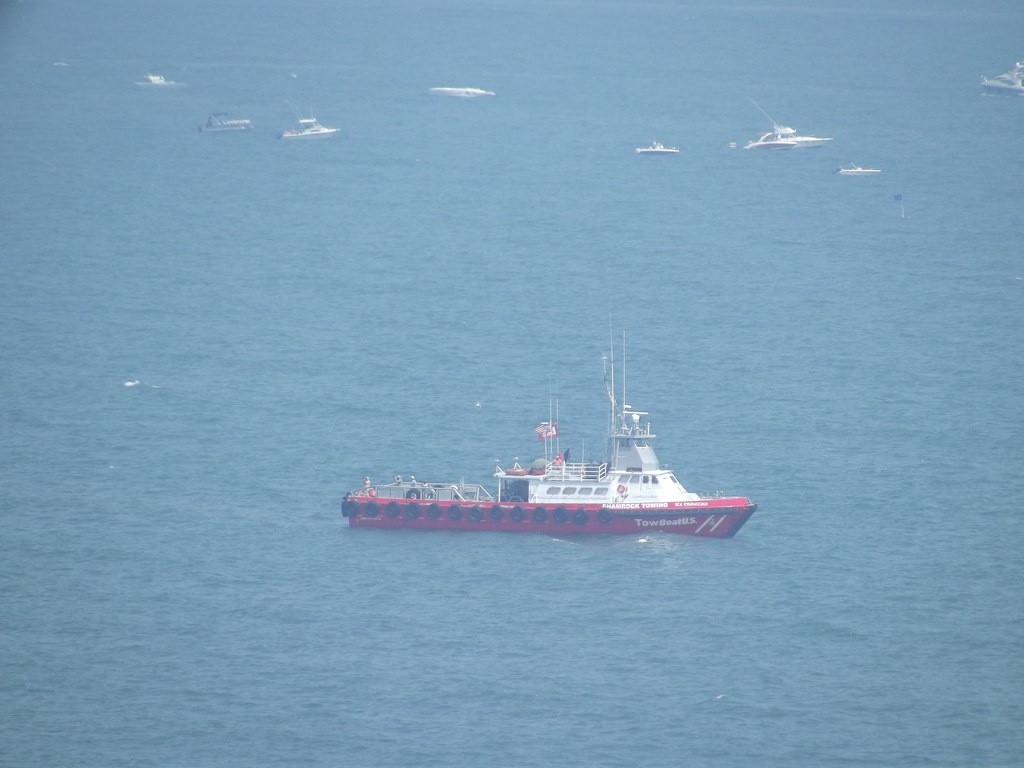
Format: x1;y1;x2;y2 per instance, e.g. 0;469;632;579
532;419;558;440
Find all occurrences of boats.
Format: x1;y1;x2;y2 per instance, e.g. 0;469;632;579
837;165;882;178
338;325;759;537
280;116;345;138
429;88;494;99
137;75;182;90
199;114;255;132
635;142;681;155
741;123;837;150
979;58;1023;95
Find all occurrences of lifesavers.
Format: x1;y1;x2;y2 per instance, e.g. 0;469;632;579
489;505;503;520
510;506;525;521
448;505;462;519
428;503;441;517
366;502;379;516
346;501;359;516
406;489;420;500
405;503;420;518
598;508;612;522
468;507;483;522
554;506;568;523
534;507;547;521
616;484;626;493
386;502;400;517
573;509;588;524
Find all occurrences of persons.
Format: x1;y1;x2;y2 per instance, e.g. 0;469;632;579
408;476;416;487
395;476;403;486
553;446;571;471
363;476;370;489
